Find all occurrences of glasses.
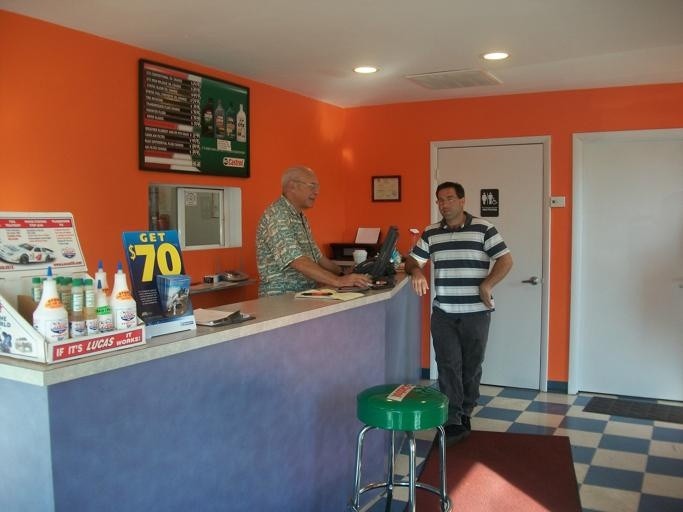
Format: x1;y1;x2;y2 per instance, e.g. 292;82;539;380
290;178;319;189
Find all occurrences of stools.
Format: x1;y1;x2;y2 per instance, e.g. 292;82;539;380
348;383;453;511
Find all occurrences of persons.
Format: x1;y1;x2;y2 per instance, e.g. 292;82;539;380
405;182;513;448
482;191;496;205
255;166;372;298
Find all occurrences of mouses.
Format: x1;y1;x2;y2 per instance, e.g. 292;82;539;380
366;281;374;289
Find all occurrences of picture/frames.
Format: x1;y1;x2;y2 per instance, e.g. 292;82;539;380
371;175;401;202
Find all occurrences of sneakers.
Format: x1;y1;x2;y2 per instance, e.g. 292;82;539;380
441;414;473;445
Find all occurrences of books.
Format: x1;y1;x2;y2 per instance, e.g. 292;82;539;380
294;290;364;300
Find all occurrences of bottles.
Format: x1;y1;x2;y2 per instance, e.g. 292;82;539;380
26;258;139;349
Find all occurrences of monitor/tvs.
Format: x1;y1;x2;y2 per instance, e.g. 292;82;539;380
369;225;399;275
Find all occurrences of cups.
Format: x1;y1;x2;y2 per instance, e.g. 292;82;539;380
352;249;368;266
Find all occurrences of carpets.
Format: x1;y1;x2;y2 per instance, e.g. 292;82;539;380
581;396;683;425
401;427;583;512
